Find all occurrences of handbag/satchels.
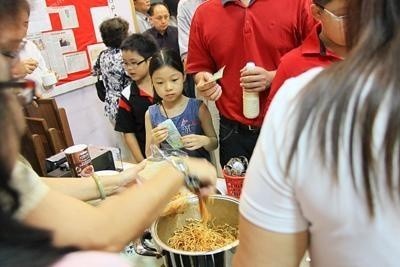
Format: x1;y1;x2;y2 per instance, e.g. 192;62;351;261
95;79;106;102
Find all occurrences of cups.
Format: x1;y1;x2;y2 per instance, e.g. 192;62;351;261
40;72;58;86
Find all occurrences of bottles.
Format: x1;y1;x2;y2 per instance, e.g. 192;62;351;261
241;60;261;118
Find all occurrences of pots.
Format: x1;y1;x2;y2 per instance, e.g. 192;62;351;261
134;191;245;266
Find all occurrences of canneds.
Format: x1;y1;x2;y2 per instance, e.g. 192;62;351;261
64;144;94;177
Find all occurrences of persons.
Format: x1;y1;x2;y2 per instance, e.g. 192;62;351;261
0;0;400;267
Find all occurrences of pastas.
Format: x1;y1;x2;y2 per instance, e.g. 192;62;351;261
167;217;239;252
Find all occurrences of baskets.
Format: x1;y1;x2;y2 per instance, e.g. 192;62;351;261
223;170;245;199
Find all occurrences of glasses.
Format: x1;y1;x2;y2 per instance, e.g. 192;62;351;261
0;77;36;106
315;3;347;28
120;56;151;68
0;40;28;67
